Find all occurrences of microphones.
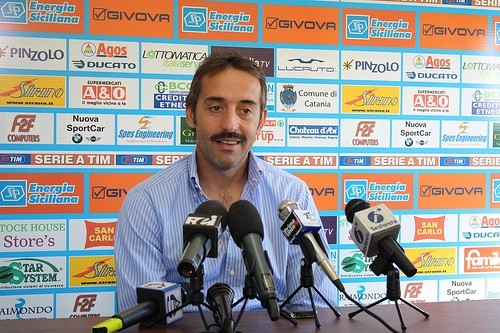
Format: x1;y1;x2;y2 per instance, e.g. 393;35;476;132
345;198;417;279
91;281;186;333
227;199;280;322
176;199;228;278
278;199;345;293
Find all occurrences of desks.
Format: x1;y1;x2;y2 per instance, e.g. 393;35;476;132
0;298;500;333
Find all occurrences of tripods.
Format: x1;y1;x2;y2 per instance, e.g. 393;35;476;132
349;238;430;331
279;231;341;327
231;250;299;333
148;238;213;333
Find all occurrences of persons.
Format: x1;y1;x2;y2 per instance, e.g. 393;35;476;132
115;53;338;314
